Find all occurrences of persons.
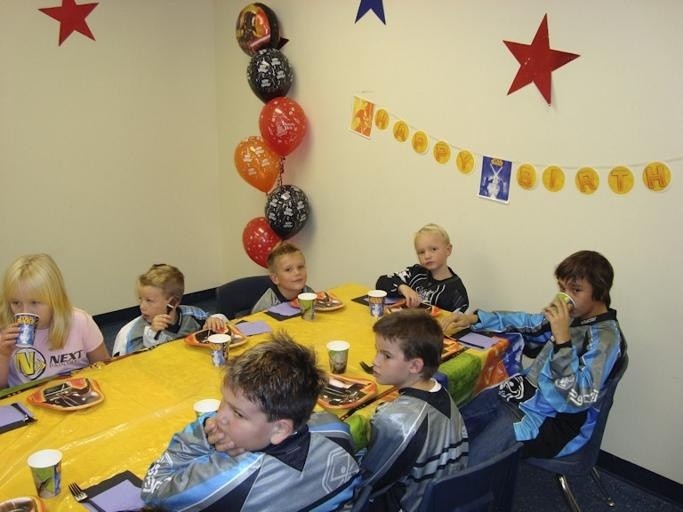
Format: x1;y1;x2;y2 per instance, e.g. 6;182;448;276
374;222;470;314
136;325;361;511
250;243;316;314
124;260;229;354
0;252;112;393
443;247;625;468
354;308;472;508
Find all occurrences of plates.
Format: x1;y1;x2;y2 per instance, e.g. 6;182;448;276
385;297;442;324
315;373;377;410
185;326;249;348
0;494;46;512
25;376;105;413
291;295;345;311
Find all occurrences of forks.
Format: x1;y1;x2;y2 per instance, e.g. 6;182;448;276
357;360;372;375
67;480;104;512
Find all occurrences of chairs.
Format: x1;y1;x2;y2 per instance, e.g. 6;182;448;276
416;442;525;512
111;315;141;357
213;273;274;324
523;351;629;512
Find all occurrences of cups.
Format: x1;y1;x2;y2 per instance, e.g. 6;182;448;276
326;340;350;374
13;312;40;348
367;290;387;317
192;398;220;419
297;292;317;320
25;448;63;500
206;333;230;366
544;292;576;322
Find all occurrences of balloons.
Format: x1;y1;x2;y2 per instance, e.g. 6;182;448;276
233;2;311;271
